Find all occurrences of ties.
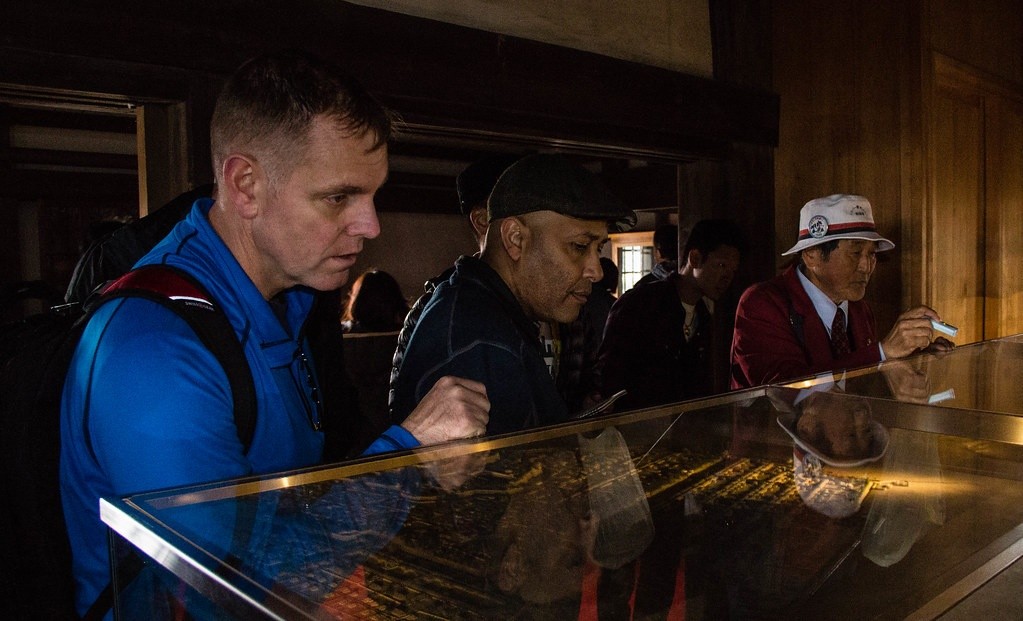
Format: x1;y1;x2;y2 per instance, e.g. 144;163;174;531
831;309;851;362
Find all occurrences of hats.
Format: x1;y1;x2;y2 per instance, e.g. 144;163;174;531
456;151;524;215
777;412;890;520
781;193;895;258
487;153;637;234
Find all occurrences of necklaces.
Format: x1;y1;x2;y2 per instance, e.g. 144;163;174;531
285;352;322;430
684;315;694;336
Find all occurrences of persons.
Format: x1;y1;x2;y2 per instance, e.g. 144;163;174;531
58;53;489;620
564;257;619;420
393;153;637;436
596;219;744;621
729;192;956;391
766;359;930;518
635;223;678;288
341;269;410;448
387;164;506;427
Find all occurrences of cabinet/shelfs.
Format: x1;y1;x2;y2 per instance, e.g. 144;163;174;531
94;328;1023;621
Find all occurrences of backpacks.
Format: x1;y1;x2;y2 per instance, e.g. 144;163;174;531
0;265;258;621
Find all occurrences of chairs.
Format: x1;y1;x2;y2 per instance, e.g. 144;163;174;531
340;332;398;427
